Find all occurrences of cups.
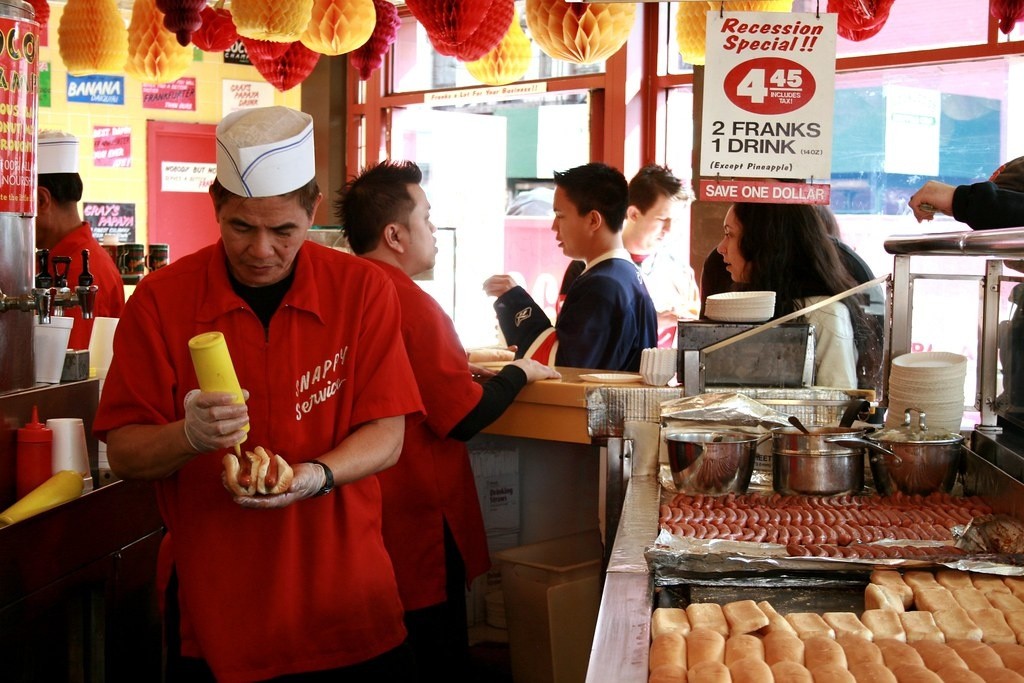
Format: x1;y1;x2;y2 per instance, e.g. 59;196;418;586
145;244;169;275
33;315;120;494
117;245;125;276
123;243;145;275
104;233;118;245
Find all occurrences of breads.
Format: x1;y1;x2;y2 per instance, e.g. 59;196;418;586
648;571;1024;683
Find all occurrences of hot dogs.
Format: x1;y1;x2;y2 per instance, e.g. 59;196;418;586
222;450;261;496
466;345;517;362
254;446;294;494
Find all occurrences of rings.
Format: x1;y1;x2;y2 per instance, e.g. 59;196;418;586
911;196;913;200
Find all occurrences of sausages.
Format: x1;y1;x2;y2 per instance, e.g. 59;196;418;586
659;490;992;560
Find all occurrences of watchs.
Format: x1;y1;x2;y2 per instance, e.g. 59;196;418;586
300;458;335;498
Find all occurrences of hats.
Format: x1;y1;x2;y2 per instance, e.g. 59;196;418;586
215;105;315;196
36;136;78;174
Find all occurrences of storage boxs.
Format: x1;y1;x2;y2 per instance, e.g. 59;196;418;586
489;532;602;683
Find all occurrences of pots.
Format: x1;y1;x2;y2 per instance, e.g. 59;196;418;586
770;426;866;497
824;407;965;498
665;428;759;495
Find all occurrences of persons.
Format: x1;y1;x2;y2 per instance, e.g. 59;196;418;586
700;202;881;391
35;133;125;319
329;156;561;682
905;155;1023;458
90;106;428;683
556;163;702;349
483;161;659;375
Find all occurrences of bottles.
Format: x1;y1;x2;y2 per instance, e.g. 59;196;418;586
188;331;251;458
17;406;51;501
0;469;88;525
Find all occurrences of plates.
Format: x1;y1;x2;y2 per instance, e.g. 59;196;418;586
705;290;776;323
639;347;676;386
883;351;967;436
578;373;642;383
